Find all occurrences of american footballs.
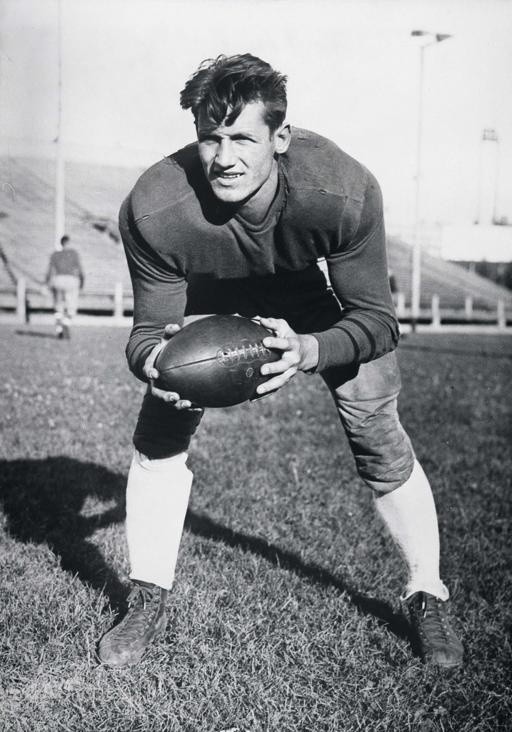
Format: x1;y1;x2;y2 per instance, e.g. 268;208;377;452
154;315;281;407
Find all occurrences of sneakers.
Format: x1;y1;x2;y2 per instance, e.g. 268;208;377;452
56;323;72;339
97;579;168;669
400;584;465;669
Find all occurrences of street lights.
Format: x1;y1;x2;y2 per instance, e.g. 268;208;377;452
410;30;452;315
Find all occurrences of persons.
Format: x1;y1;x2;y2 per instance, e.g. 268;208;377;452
96;53;464;668
46;236;84;339
